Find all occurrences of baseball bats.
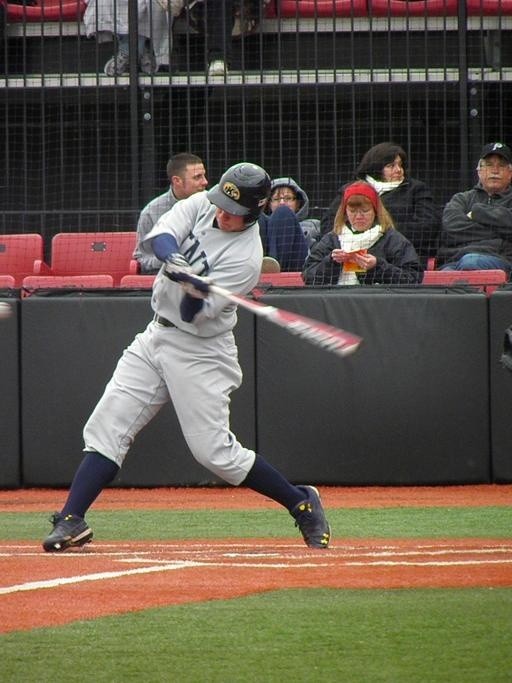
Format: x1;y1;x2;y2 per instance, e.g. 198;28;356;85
209;281;362;356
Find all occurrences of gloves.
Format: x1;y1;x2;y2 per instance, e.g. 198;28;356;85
166;253;192;281
182;275;214;300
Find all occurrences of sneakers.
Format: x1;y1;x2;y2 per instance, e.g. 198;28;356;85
105;42;130;78
289;485;330;549
43;511;93;550
208;60;228;77
139;44;159;78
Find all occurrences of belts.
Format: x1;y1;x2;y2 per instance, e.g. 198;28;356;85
153;314;174;327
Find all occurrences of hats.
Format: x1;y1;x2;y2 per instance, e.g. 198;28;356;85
480;143;512;165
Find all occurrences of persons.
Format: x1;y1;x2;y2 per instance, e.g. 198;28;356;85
435;141;511;272
322;142;439;270
299;182;425;285
83;0;188;76
258;177;322;274
132;151;209;274
43;160;330;553
181;0;268;74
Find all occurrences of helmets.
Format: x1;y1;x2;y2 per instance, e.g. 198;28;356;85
207;163;272;223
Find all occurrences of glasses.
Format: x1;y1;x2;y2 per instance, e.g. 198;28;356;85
271;194;296;204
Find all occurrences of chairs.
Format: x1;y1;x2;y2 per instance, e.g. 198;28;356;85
423;257;507;287
1;2;90;22
263;2;510;21
1;232;45;288
255;271;308;289
119;259;156;288
20;232;141;288
